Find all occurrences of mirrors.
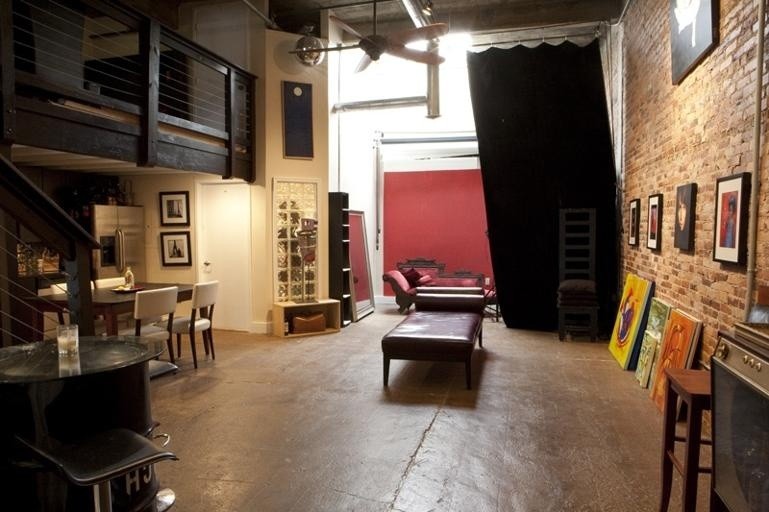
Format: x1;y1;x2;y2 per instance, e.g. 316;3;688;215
343;209;376;324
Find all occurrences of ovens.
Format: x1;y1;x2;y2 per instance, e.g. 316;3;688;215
710;335;769;512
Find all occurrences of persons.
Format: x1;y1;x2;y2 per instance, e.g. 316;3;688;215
676;190;688;231
723;195;735;247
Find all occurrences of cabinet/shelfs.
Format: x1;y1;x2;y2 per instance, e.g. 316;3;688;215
271;297;341;339
327;190;354;329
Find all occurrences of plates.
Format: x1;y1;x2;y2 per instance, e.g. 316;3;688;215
110;285;145;292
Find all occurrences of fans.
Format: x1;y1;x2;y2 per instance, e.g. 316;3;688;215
286;0;450;74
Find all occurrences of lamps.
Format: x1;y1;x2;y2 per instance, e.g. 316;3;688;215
403;0;442;46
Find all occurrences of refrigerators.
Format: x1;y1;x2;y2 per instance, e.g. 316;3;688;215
90;204;147;283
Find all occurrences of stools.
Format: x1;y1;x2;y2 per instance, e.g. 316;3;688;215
14;422;182;512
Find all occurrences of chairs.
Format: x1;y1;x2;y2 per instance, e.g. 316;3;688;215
158;280;222;371
50;278;128;335
95;277;162;328
117;286;180;383
553;200;603;344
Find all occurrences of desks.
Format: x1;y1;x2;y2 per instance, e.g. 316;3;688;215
0;330;172;512
658;366;714;512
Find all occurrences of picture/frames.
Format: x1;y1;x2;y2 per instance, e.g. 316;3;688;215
645;191;663;253
160;230;193;267
158;189;192;226
712;171;752;270
628;196;641;247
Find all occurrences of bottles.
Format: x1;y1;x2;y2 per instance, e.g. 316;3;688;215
32;257;38;274
125;266;134;288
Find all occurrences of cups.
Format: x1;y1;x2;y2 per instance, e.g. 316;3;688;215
37;259;44;275
57;323;79;356
58;355;81;377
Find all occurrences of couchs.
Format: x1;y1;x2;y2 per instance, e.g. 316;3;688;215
381;259;500;393
